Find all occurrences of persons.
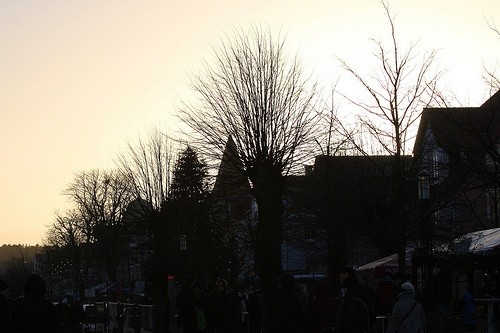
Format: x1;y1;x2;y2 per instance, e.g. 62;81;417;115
2;252;500;333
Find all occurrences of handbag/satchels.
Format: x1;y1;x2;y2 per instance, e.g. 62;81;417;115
394;326;400;333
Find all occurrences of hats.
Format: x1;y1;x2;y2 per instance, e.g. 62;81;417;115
401;282;414;291
